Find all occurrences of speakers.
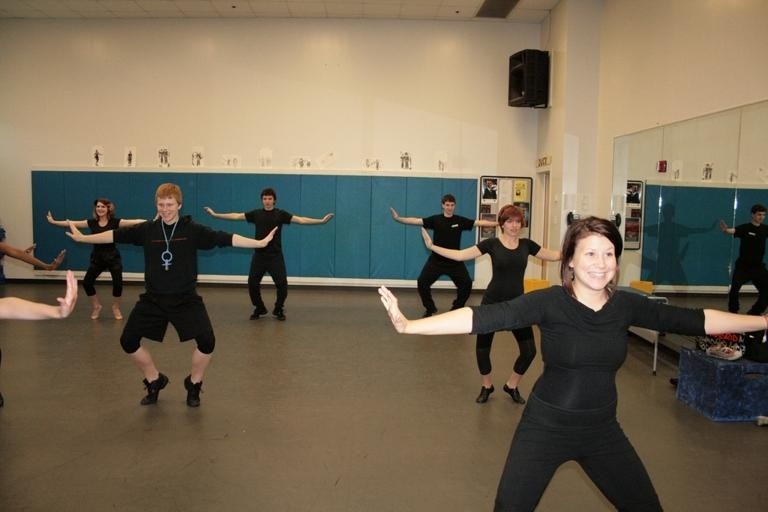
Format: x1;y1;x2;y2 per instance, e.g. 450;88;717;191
508;49;551;109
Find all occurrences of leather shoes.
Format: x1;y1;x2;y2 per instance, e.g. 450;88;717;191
502;383;525;404
476;384;495;403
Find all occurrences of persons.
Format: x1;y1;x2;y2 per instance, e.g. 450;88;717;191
1;269;80;322
46;197;160;321
203;187;336;321
483;180;497;198
0;242;67;269
24;243;37;253
375;210;768;512
62;183;278;407
420;203;563;406
719;203;768;316
389;193;499;317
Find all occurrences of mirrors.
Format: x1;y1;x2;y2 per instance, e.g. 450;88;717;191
609;99;768;317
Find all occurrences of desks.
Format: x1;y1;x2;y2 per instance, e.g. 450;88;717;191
676;345;768;422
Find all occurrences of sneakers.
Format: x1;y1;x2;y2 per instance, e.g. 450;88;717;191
272;307;285;321
184;375;203;407
91;304;102;319
140;373;168;406
111;307;122;320
249;306;268;320
422;305;437;317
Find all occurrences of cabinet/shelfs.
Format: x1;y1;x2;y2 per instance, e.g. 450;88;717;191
639;177;768;295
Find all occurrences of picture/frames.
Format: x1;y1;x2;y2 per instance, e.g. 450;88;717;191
478;175;533;245
622;179;644;251
